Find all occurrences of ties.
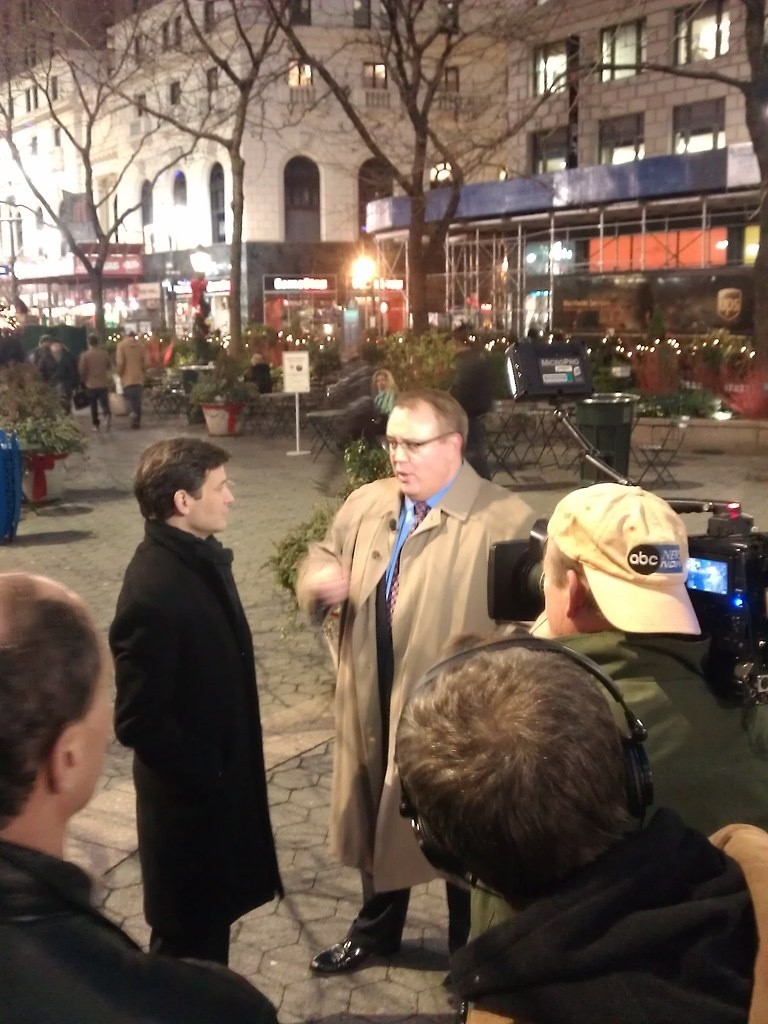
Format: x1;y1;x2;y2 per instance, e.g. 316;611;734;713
385;501;431;636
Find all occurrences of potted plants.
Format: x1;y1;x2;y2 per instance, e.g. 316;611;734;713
10;420;86;504
186;351;257;436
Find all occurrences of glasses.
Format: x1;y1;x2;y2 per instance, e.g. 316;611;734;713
381;431;457;453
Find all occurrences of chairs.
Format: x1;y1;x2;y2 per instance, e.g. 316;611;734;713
151;385;693;490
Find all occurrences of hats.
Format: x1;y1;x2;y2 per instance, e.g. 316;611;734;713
548;482;704;635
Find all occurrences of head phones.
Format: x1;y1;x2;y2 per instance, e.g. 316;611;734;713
391;637;655;888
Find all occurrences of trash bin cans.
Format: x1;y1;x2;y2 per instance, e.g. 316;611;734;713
577;393;641;487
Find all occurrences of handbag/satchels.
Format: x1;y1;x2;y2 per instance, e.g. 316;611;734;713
71;387;92;411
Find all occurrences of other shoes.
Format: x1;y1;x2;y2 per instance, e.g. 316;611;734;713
91;424;100;431
129;412;139;430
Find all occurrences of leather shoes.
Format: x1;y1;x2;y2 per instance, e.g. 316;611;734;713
455;999;468;1024
310;936;392;975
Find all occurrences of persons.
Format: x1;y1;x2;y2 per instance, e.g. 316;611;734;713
392;634;760;1024
465;479;768;944
448;326;496;481
295;388;541;1015
0;573;281;1024
244;352;274;394
693;564;724;593
108;439;282;970
26;331;148;429
336;369;399;454
